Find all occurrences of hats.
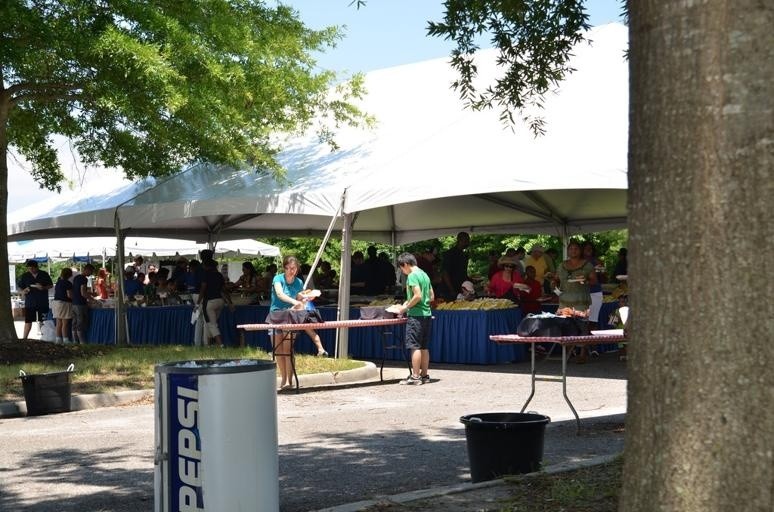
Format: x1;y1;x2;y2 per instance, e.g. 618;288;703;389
497;257;519;269
461;281;475;295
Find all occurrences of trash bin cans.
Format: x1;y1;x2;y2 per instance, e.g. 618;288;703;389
153;359;280;512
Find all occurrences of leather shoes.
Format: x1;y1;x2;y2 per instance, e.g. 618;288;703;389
576;356;585;364
592;350;600;358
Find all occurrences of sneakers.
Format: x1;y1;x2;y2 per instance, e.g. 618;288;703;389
408;374;430;383
399;376;422;385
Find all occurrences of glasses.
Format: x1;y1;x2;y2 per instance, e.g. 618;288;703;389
505;264;513;268
284;266;296;272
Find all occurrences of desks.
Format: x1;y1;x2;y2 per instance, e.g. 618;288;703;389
483;329;629;437
234;315;413;393
46;284;625;369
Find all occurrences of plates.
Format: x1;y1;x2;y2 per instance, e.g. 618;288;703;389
29;285;44;288
567;279;586;283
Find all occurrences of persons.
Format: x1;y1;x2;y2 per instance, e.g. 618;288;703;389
265;256;304;390
398;251;435;385
